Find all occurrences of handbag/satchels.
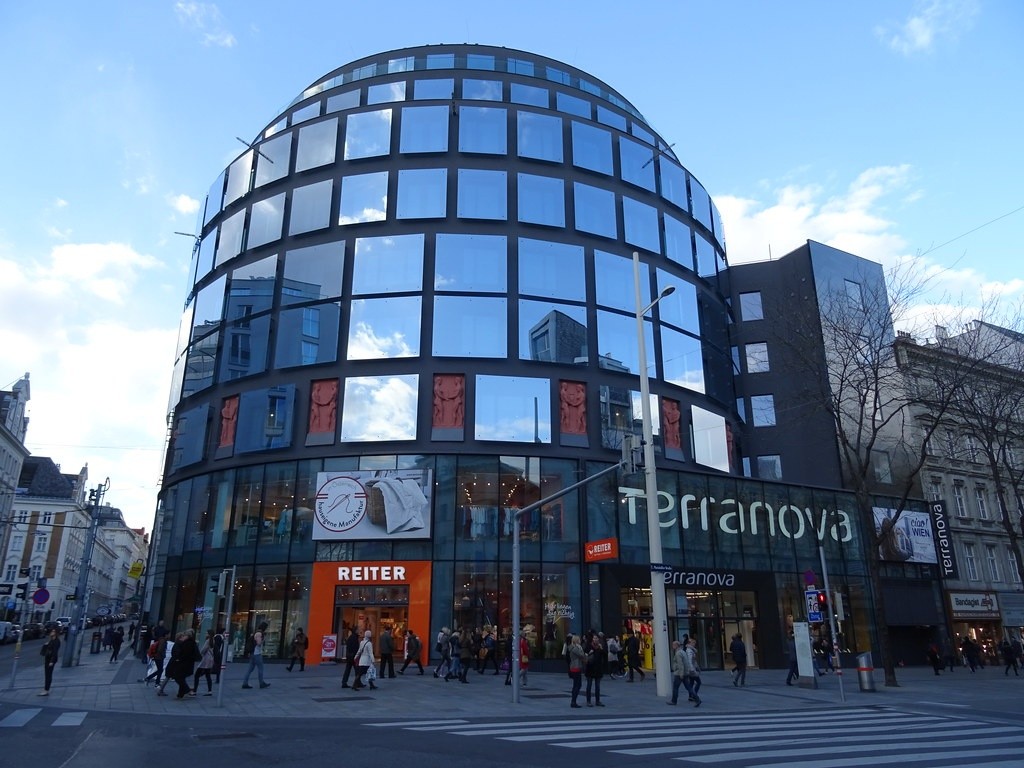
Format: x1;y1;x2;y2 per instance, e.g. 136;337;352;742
354;655;361;666
435;642;442;652
365;664;376;681
40;645;48;656
522;655;528;662
167;655;191;678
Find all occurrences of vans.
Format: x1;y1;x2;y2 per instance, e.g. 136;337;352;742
0;622;12;642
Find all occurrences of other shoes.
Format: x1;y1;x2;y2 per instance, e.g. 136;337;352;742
492;672;499;675
571;703;581;708
242;684;253;688
143;678;213;698
287;666;292;672
37;689;49;696
596;702;605;706
477;671;483;675
819;673;825;676
609;674;703;707
732;680;739;687
396;670;404;675
587;703;592;706
342;675;396;690
260;683;269;688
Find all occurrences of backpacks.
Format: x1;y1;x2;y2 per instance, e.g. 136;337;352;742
416;639;423;652
246;631;263;652
149;641;159;657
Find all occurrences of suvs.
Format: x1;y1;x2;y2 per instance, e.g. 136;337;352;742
56;616;72;631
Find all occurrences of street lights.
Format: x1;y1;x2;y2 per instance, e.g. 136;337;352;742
637;286;676;696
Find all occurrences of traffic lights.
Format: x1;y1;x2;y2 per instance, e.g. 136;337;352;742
15;581;30;601
209;571;227;596
818;592;827;612
834;592;851;621
621;434;647;476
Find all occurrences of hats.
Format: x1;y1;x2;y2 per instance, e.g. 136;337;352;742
365;631;371;637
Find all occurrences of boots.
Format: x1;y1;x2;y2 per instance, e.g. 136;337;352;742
433;668;469;683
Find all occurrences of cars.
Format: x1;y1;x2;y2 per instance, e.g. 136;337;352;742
79;613;140;629
12;620;62;644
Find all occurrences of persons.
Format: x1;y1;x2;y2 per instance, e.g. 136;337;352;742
785;630;836;686
379;626;396;679
665;633;702;708
242;622;271;689
1002;638;1024;676
562;629;645;683
141;620;170;665
926;634;986;676
569;634;607;709
729;632;748;688
143;629;172;690
286;627;307;672
157;628;228;700
396;630;425;675
624;619;653;654
342;625;378;691
504;630;533;688
128;621;135;641
103;623;124;664
37;628;61;696
433;624;500;684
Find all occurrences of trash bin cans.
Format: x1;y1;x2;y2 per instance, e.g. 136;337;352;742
856;651;876;692
91;632;102;655
